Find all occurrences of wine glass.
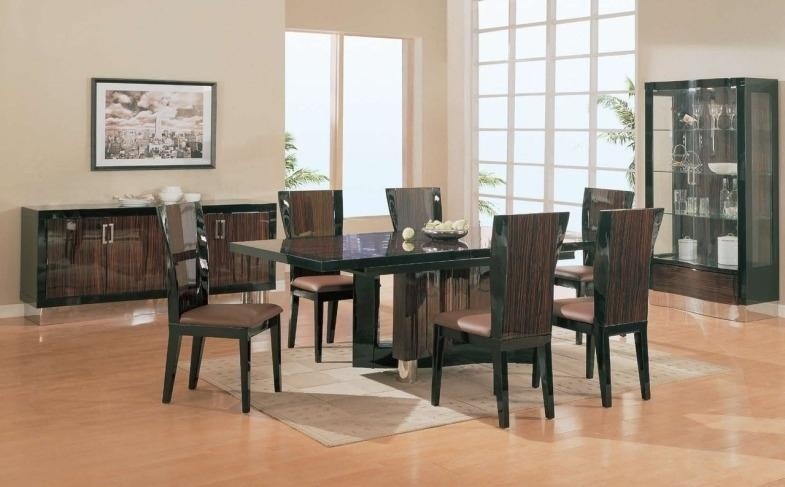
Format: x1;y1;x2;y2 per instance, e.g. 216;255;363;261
691;101;737;132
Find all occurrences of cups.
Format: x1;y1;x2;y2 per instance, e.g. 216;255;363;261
673;189;708;216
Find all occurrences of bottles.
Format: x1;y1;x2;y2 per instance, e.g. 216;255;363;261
726;179;739;219
719;179;732;218
672;107;703;128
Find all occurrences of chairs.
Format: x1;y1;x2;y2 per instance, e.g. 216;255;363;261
156;203;283;413
278;190;379;363
533;208;665;408
387;188;443;232
554;188;635;344
432;212;570;427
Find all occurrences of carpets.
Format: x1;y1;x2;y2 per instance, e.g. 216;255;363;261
177;338;721;448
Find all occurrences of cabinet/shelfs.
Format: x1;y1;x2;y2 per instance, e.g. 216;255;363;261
644;79;778;305
20;200;277;328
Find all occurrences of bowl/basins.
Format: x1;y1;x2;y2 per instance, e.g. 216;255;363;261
421;226;468;240
708;163;738;177
156;185;183;206
421;242;467;254
183;193;202;202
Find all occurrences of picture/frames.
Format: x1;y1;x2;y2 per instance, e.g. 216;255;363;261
91;78;217;169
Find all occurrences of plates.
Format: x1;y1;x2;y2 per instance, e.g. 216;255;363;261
115;197;151;208
